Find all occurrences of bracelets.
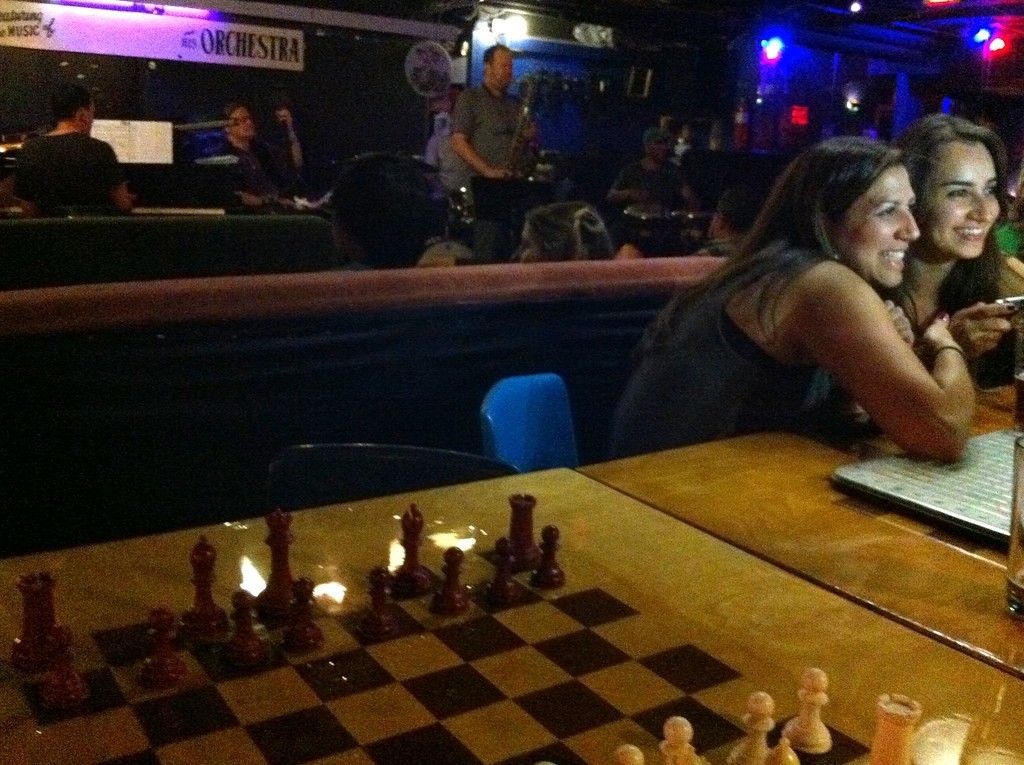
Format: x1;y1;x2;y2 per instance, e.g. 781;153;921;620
932;341;965;360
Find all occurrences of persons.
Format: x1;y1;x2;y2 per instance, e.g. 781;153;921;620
425;85;469;191
605;137;975;461
453;45;536;264
510;202;615;263
608;125;702;260
886;114;1024;389
331;151;437;270
225;104;305;208
692;186;758;257
10;82;134;216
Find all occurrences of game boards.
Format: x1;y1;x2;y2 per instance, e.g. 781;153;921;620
1;533;917;765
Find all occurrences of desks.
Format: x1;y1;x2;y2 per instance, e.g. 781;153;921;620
1;371;1022;765
574;365;1022;675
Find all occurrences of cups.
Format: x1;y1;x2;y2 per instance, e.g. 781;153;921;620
1004;435;1023;621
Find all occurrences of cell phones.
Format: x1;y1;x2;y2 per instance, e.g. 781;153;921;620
993;297;1024;320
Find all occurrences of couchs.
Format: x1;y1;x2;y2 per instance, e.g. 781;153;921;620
1;203;734;545
